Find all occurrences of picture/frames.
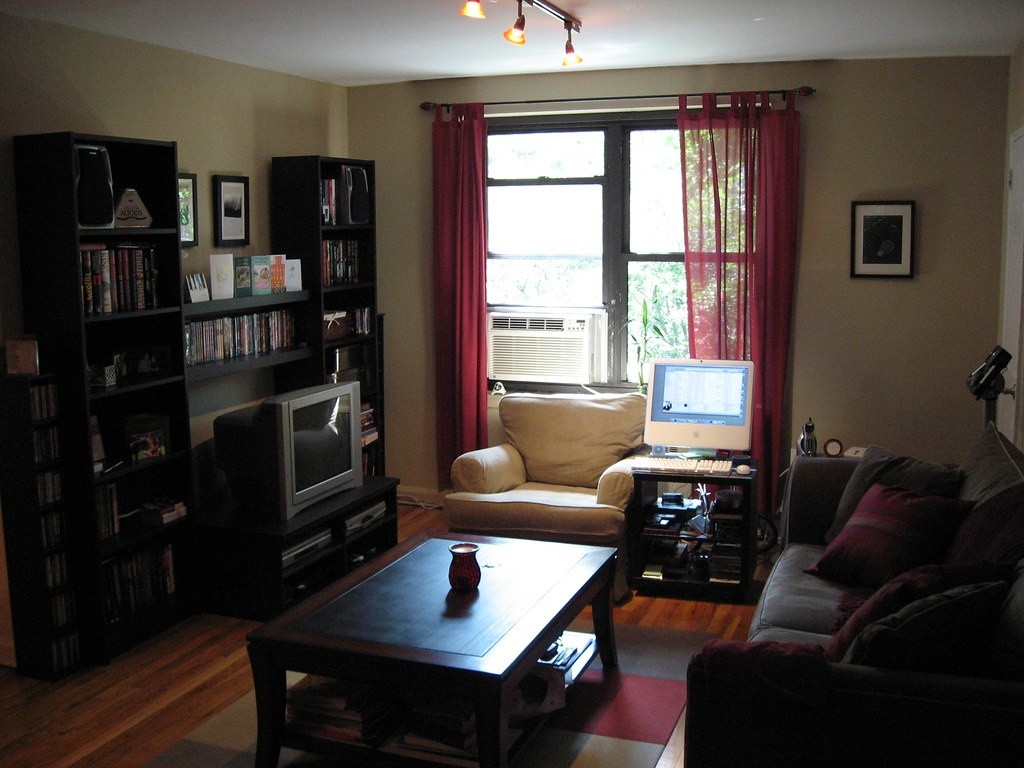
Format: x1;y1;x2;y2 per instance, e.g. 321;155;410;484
850;200;916;279
178;172;199;248
213;175;250;247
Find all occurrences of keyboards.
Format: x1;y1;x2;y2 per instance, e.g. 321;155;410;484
630;456;733;477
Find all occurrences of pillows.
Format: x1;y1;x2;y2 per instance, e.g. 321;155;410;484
802;442;1005;679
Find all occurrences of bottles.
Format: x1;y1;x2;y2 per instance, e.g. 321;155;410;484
449;543;481;593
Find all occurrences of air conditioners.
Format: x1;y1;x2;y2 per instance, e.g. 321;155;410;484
486;308;606;384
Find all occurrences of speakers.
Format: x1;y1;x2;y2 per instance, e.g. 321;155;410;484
73;143;117;230
339;165;371;228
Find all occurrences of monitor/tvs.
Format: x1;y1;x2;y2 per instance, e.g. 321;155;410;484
643;358;755;451
213;381;363;523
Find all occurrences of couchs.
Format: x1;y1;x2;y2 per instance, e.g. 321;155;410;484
445;392;647;607
686;420;1024;768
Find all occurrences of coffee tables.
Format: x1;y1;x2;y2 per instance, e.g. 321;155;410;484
247;529;619;768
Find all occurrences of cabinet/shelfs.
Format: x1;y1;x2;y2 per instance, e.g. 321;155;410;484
0;131;400;683
632;468;758;603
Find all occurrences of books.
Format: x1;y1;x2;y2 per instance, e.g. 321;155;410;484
641;510;744;584
285;636;593;761
31;176;379;676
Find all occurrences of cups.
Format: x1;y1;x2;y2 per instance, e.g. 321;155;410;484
732;454;752;467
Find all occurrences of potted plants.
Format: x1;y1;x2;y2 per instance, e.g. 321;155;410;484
619;298;669;395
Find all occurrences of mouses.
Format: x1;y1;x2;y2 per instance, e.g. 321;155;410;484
736;465;751;474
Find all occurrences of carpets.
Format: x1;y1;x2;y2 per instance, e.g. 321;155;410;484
139;620;719;767
